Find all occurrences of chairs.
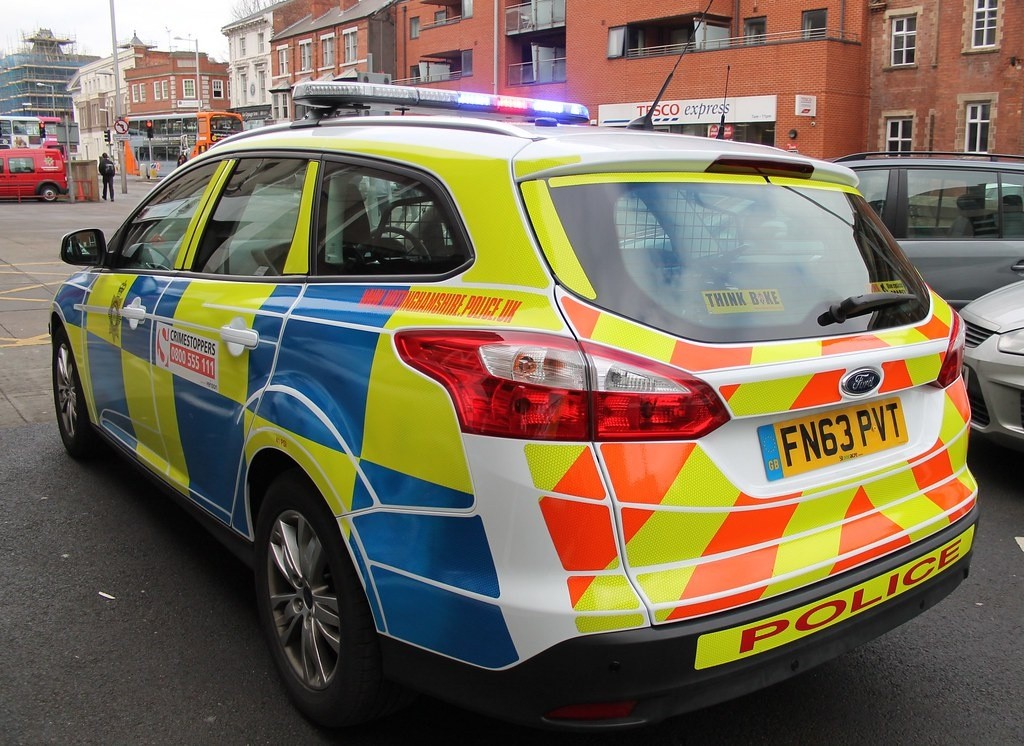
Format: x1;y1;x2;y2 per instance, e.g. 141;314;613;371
316;182;371;270
946;195;979;238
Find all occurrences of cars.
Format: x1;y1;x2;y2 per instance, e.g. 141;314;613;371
953;195;957;197
957;257;1024;469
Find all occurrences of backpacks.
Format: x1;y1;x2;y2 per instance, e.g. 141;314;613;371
104;159;115;176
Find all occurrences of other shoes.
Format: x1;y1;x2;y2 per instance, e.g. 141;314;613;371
102;195;107;200
111;199;114;201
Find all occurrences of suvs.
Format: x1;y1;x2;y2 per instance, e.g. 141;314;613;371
49;79;980;732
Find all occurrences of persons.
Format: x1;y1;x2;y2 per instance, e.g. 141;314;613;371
99;153;114;201
177;155;187;167
395;185;419;227
365;177;391;228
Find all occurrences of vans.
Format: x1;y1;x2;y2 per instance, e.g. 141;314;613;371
0;149;70;203
816;150;1024;313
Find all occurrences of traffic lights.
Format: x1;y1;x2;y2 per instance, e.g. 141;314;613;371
147;121;153;139
39;122;46;138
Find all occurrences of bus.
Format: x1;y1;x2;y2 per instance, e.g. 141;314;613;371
115;109;245;179
1;115;67;153
